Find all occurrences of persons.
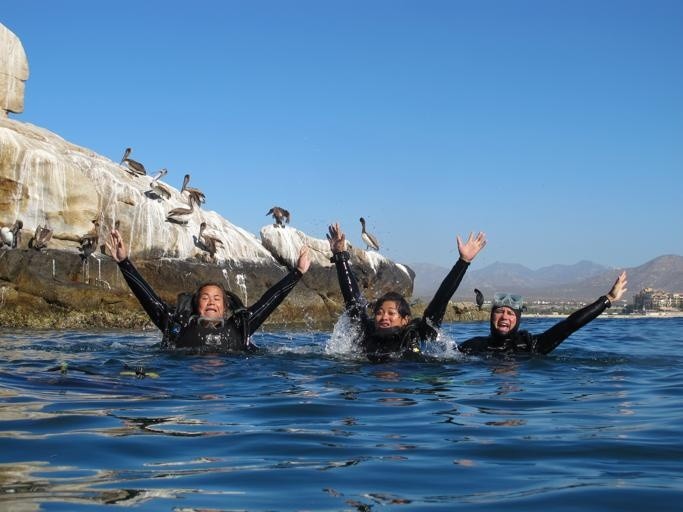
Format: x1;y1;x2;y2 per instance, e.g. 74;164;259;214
103;229;314;357
323;223;488;351
455;270;627;353
